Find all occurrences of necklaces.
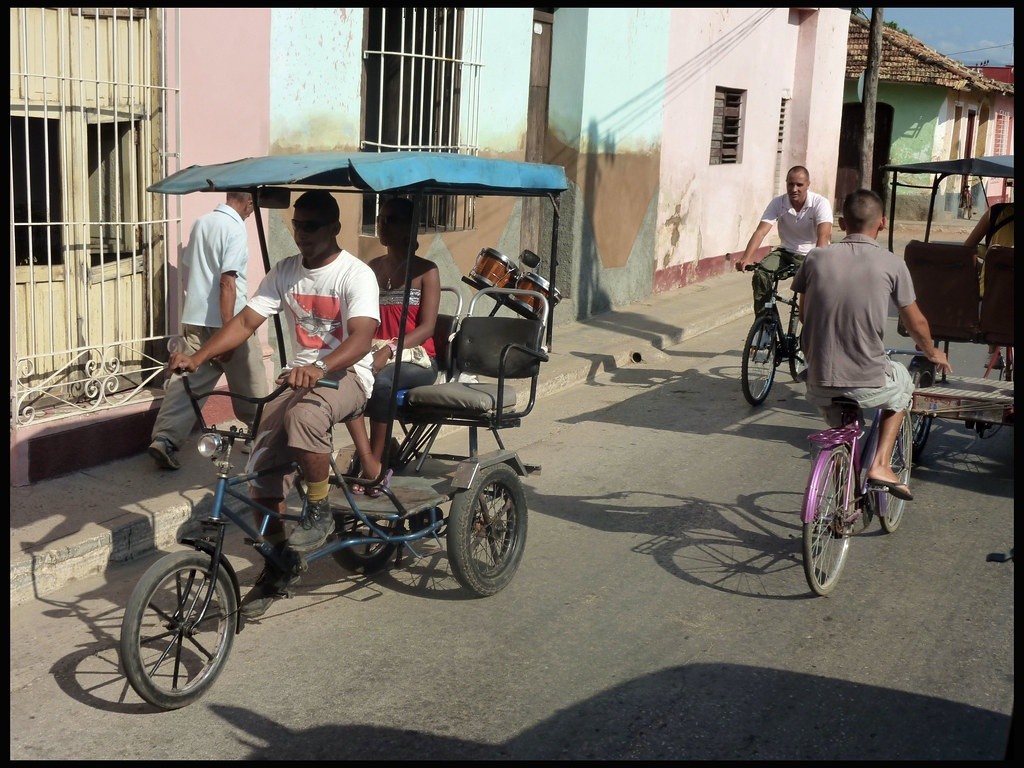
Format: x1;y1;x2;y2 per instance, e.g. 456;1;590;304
383;259;407;289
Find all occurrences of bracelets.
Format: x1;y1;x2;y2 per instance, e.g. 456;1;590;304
386;343;397;359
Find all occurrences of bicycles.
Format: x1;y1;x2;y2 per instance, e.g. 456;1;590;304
738;258;807;406
798;348;928;596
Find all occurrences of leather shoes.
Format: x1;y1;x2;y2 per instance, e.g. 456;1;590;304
288;497;336;550
239;540;308;619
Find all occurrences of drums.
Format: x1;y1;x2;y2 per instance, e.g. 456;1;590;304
469;246;564;321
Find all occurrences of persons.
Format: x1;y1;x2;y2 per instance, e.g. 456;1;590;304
736;166;833;340
343;198;441;498
790;188;953;539
172;190;381;618
965;201;1024;369
148;192;269;470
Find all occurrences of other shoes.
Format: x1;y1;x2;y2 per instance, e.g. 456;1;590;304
984;351;1004;369
799;367;808;382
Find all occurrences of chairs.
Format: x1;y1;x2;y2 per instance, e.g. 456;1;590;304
897;239;1015;374
394;286;550;472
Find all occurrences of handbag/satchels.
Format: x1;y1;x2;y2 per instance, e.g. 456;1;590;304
371;338;432;369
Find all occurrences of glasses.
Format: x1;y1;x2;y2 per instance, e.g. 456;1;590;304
291;217;331;233
376;213;407;225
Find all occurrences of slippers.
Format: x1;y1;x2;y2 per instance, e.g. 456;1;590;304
367;466;393;496
352;470;368;494
866;477;913;502
824;513;843;539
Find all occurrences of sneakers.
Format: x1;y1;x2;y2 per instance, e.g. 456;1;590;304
241;427;254;453
148;437;181;469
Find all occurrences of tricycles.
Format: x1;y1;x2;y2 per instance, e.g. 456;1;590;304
118;149;571;712
876;155;1013;468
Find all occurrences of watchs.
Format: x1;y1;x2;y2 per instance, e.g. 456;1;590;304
312;360;328;378
